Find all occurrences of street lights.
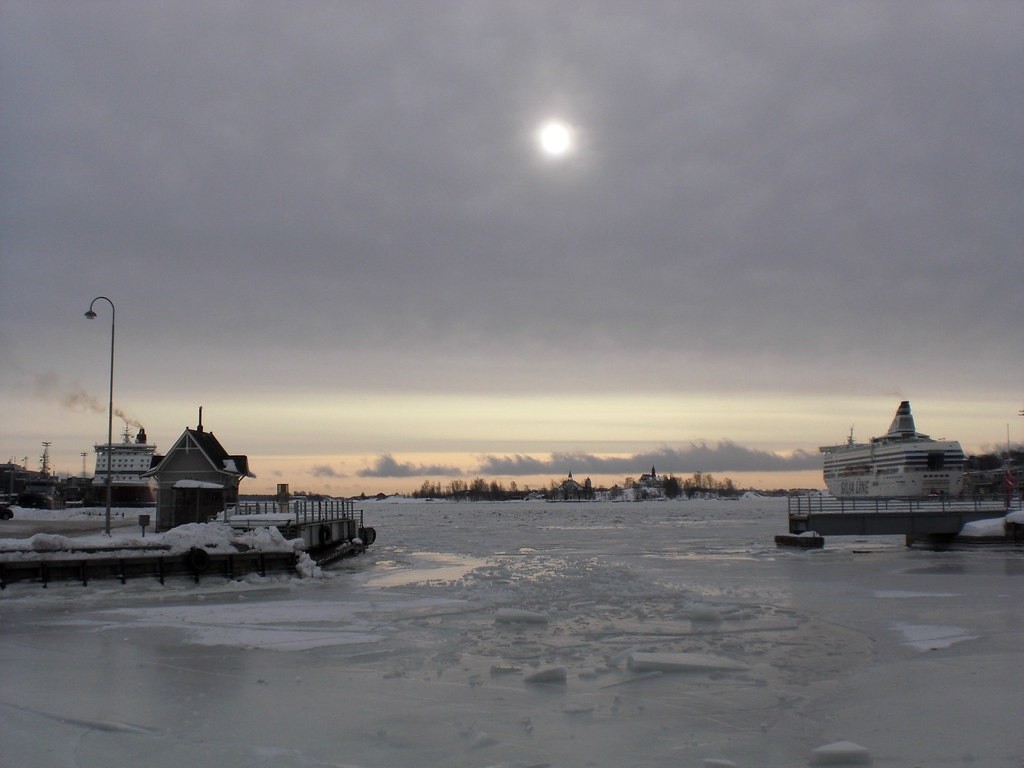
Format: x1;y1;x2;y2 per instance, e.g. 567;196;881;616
82;295;116;536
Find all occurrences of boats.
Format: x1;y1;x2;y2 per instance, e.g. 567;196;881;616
16;439;67;511
63;450;92;502
85;430;160;506
820;401;966;501
774;532;823;550
